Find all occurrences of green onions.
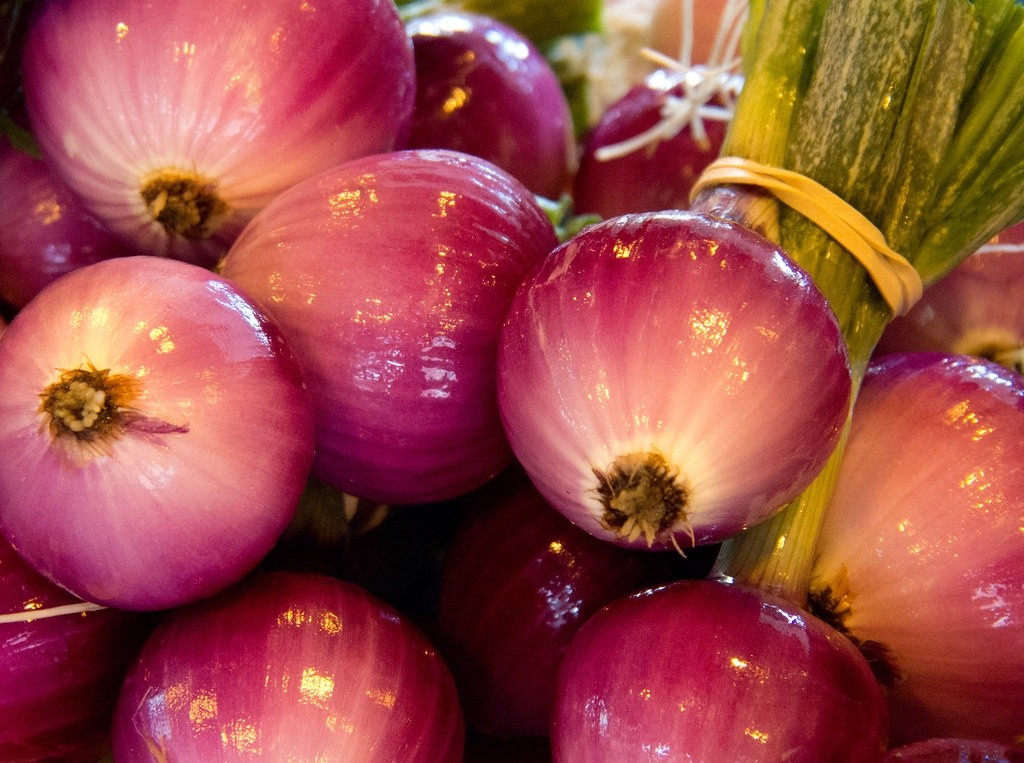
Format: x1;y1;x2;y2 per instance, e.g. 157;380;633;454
672;0;1024;612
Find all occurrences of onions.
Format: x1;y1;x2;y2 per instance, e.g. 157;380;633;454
0;0;1024;763
